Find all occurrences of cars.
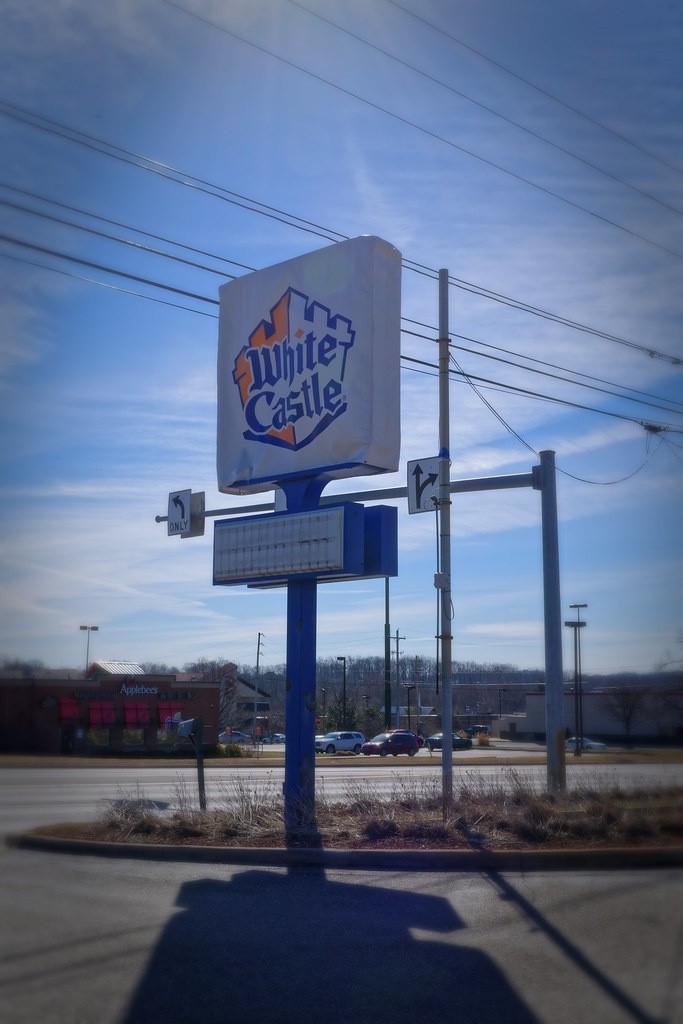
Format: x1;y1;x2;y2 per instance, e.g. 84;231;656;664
261;734;286;745
565;737;606;751
217;731;252;743
425;732;472;752
361;730;420;758
469;726;491;735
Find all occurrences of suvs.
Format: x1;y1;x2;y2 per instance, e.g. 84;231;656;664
315;731;366;754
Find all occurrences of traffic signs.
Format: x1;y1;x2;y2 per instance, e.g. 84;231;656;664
166;488;195;537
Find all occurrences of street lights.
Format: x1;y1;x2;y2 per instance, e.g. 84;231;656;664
80;624;99;677
569;603;589;752
564;620;587;754
499;688;507;718
337;656;352;731
